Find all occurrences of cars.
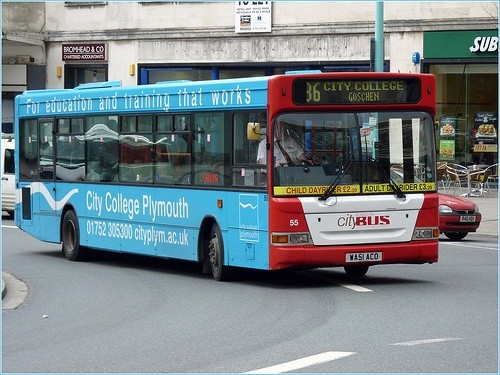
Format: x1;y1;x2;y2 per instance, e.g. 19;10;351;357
438;192;481;240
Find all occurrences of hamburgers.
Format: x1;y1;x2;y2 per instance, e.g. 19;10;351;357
477;124;493;136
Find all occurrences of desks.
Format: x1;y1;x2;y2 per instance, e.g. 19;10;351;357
467;165;489;193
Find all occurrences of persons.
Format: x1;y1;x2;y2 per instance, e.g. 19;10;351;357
257;122;315;173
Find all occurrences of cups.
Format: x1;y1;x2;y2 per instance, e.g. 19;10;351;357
473;165;478;170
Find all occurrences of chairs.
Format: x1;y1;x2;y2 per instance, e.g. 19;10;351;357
437;161;498;197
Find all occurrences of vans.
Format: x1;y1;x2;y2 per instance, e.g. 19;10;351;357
1;137;15;220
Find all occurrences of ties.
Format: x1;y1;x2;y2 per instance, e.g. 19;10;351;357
275;139;293;167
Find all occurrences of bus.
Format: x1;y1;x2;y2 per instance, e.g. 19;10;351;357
14;69;438;280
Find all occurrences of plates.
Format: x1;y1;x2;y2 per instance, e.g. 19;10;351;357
441;128;455;134
478;128;496;137
